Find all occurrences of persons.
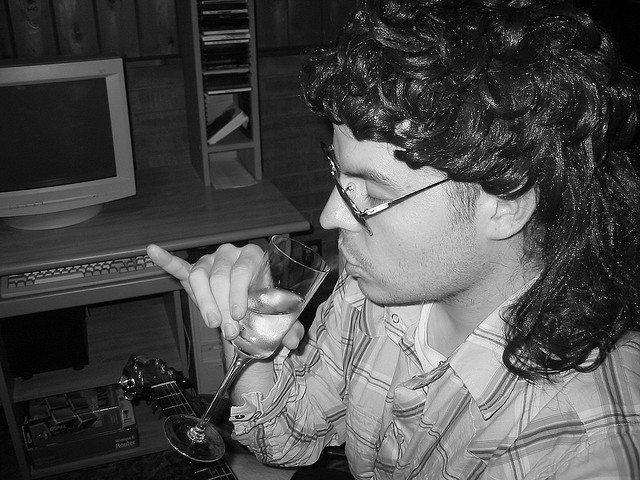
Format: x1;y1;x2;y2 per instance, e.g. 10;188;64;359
147;0;640;480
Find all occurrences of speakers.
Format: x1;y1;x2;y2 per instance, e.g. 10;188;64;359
1;305;90;380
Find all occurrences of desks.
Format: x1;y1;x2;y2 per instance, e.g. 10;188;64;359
2;159;310;395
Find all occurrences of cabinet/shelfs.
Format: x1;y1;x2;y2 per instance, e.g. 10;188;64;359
190;0;263;192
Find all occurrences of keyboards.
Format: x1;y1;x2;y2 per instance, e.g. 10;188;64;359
2;250;189;300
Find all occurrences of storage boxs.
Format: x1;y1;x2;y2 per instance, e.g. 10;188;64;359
12;383;142;471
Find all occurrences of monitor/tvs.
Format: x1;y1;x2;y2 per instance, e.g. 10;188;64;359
1;55;137;229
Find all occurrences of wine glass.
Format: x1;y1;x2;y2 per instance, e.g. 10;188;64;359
162;234;330;463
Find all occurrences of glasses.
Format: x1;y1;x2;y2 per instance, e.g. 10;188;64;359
318;140;451;237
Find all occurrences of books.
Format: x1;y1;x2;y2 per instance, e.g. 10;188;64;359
197;0;252;95
205;106;249;145
